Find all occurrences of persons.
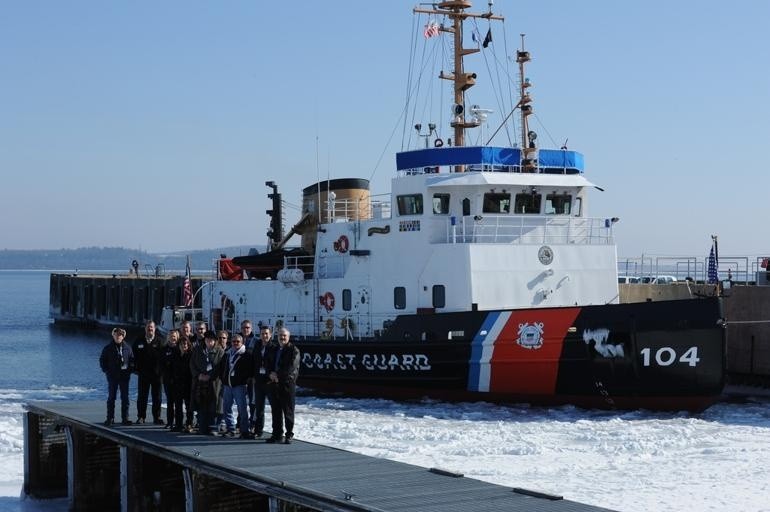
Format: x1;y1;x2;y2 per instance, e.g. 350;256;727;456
100;319;301;443
728;269;733;280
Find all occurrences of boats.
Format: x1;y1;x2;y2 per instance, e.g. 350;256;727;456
198;0;730;412
155;303;201;350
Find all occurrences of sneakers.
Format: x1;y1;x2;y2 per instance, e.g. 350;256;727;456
136;418;146;424
154;418;164;426
104;417;114;426
165;421;293;443
122;418;132;426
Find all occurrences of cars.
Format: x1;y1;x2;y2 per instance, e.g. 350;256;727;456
617;275;640;284
632;275;677;284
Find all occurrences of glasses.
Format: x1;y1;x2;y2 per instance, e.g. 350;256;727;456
195;327;250;343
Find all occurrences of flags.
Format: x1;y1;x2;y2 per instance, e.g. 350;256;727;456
708;245;718;283
423;24;439;38
482;28;493;48
183;263;192;306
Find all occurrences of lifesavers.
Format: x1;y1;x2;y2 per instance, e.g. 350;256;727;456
324;292;334;310
338;236;348;252
